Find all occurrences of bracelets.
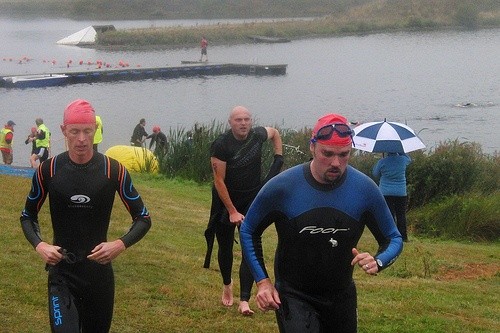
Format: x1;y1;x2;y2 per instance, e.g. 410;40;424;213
257;278;270;286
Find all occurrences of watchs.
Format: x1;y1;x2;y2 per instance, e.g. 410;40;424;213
374;257;383;273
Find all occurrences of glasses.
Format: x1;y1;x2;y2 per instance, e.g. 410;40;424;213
315;124;355;141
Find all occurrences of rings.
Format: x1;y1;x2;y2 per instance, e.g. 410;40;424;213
365;264;369;270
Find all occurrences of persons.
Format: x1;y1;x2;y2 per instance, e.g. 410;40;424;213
239;114;403;333
25;118;51;168
148;125;167;156
200;36;209;61
203;106;283;316
371;153;412;243
19;99;152;333
93;114;103;153
0;120;16;166
130;118;149;147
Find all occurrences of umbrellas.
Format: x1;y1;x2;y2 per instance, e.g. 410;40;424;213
350;117;426;158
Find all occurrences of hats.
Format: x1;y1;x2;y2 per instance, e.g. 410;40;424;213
31;127;37;132
8;121;17;126
63;99;97;124
311;113;353;147
153;126;160;132
